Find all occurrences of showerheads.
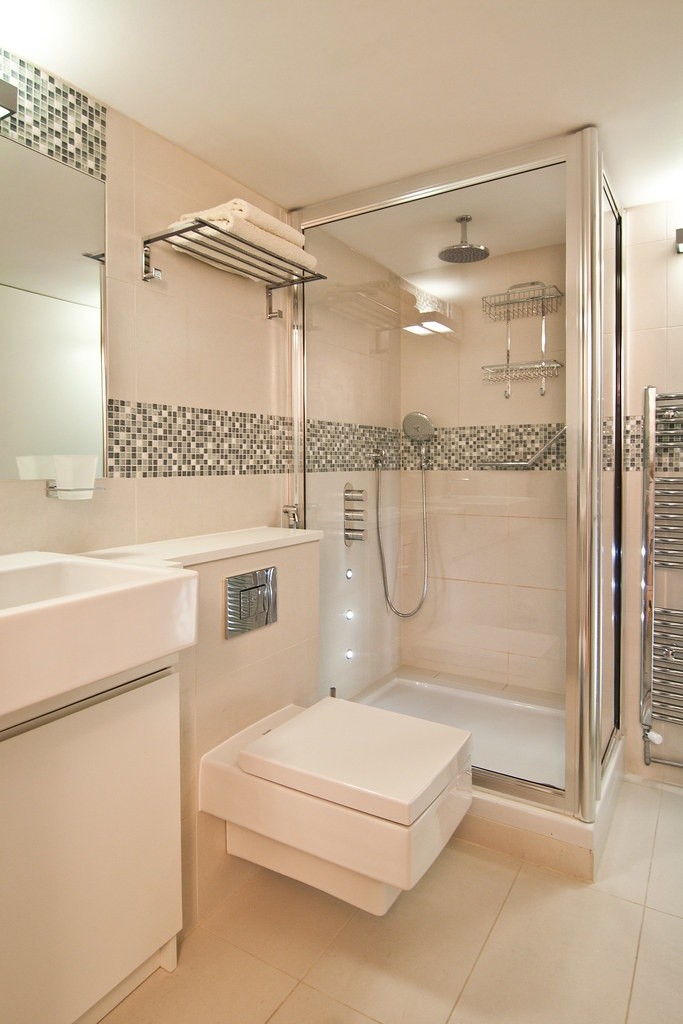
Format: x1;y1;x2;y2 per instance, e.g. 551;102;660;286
438;216;488;262
402;411;434;471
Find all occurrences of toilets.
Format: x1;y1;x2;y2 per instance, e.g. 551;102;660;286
88;517;476;918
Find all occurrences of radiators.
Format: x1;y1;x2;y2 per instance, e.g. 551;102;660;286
639;386;682;768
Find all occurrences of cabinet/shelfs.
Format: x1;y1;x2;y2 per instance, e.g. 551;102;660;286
0;667;183;1023
480;282;563;400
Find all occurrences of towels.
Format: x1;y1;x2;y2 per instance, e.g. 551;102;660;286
179;196;305;247
166;214;316;283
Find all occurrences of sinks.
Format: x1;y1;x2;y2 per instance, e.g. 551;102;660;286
0;550;196;719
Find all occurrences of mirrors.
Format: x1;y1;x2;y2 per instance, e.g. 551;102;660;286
0;139;109;484
